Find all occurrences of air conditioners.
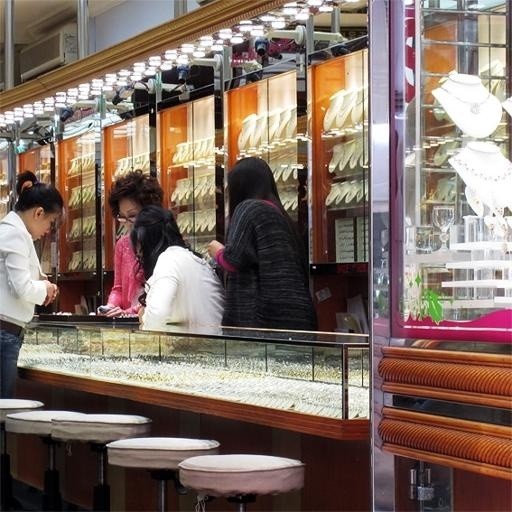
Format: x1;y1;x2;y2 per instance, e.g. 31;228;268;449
16;20;78;82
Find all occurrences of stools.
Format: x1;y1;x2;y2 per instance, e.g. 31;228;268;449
0;398;308;512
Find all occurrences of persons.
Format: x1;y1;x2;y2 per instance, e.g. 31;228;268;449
207;156;320;377
129;205;226;359
94;167;165;318
0;169;64;399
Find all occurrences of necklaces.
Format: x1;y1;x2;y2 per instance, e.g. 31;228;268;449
425;56;512;210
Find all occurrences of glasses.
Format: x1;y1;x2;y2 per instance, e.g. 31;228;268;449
117;212;139;224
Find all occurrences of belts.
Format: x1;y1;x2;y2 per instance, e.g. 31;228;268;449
0;319;26;341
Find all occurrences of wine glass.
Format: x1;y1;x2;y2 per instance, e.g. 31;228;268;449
432;207;453;254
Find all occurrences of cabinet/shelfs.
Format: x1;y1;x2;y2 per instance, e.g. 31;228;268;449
425;117;508;209
0;37;369;284
